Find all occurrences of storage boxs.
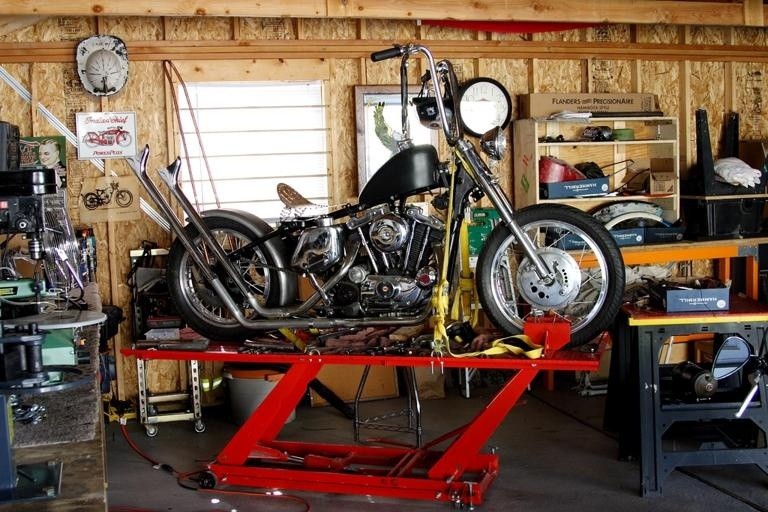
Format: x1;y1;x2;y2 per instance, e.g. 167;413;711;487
519;93;664;120
648;277;730;314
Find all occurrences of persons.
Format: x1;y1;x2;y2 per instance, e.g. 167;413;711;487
37;136;67;189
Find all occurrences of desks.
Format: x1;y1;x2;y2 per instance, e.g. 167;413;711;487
520;237;768;392
679;194;768;240
0;364;108;512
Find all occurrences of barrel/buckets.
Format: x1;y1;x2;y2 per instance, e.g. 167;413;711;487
224;366;297;425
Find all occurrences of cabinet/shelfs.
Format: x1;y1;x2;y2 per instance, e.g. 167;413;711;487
511;116;681;251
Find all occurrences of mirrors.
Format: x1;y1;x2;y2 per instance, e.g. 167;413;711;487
354;85;440;191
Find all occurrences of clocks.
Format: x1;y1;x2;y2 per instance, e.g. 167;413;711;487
455;77;513;139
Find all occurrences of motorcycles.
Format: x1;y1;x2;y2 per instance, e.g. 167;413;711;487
125;43;625;352
81;126;133;211
